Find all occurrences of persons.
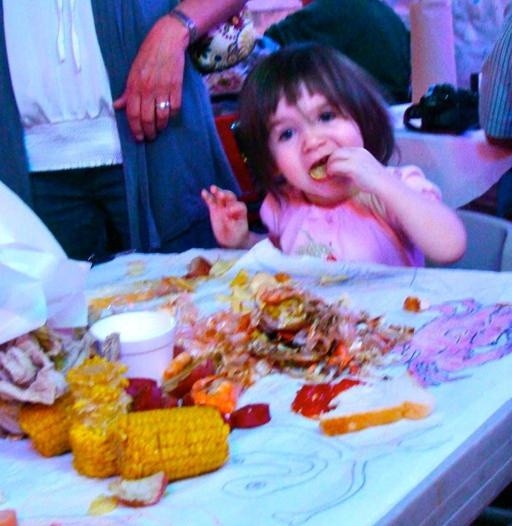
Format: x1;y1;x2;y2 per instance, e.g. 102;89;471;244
264;1;412;110
0;1;249;264
479;2;512;220
200;38;467;268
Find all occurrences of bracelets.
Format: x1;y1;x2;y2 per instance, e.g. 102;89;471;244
168;9;197;44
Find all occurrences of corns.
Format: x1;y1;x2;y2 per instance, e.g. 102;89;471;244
68;354;128;478
110;406;230;483
18;393;73;455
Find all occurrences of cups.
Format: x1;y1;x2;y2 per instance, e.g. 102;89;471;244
89;312;177;389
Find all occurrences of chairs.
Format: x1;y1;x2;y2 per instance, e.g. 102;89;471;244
427;209;512;271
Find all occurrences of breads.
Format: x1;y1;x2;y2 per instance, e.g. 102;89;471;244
320;371;433;436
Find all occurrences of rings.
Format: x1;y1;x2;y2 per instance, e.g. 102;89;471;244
156;100;171;113
